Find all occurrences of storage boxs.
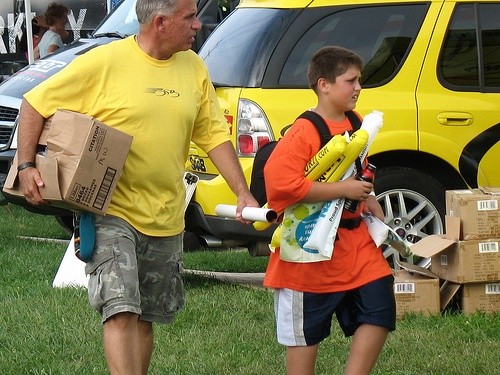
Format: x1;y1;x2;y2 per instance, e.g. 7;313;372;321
2;109;134;216
393;187;500;320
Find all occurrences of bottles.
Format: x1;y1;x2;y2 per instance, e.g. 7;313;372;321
343;163;377;213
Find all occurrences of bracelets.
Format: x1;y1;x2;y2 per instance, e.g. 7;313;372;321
17;161;36;171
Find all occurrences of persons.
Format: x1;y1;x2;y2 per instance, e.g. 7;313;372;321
26;3;70;62
264;45;397;375
17;0;261;375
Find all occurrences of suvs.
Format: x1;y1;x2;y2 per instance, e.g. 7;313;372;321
0;0;500;272
